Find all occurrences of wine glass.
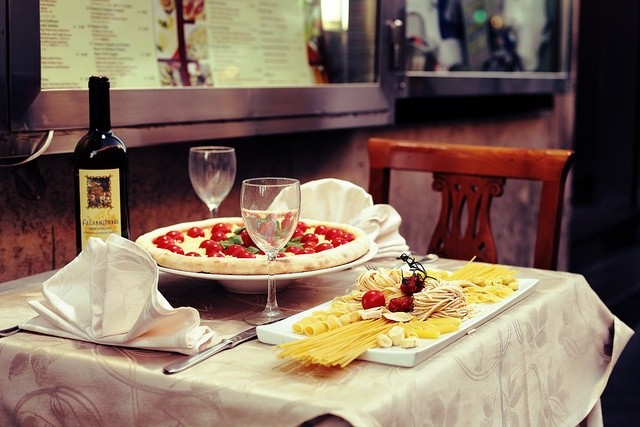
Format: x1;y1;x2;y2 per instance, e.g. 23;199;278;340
188;146;237;219
240;177;301;326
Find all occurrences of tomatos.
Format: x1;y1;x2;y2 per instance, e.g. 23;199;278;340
361;290;386;310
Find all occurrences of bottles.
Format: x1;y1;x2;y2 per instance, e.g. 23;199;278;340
72;76;131;258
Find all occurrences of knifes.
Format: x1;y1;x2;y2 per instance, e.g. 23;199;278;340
162;317;287;375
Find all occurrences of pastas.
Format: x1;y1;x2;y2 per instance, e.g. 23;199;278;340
352;268;468;319
273;258;515;369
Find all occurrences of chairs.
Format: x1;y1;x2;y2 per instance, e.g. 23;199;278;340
363;135;579;271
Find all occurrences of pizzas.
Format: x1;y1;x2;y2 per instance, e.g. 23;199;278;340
134;216;371;275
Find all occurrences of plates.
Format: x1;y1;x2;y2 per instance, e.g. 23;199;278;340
157;240;379;294
255;265;540;368
